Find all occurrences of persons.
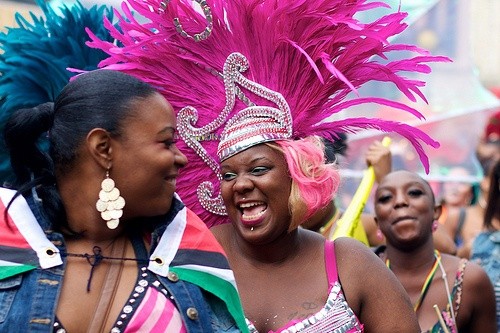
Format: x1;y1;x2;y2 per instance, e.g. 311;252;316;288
292;135;393;268
470;156;500;332
373;169;497;332
431;141;500;260
209;108;423;332
0;69;249;333
